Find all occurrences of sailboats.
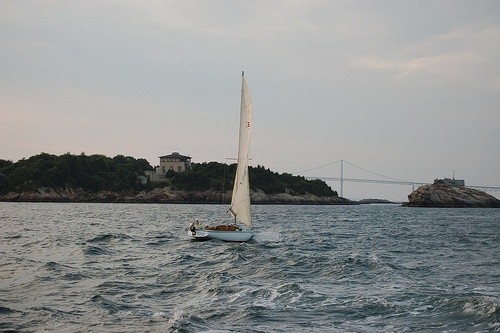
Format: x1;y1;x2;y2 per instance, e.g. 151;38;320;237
183;70;253;243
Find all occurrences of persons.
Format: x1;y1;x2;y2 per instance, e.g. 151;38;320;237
188;219;205;230
